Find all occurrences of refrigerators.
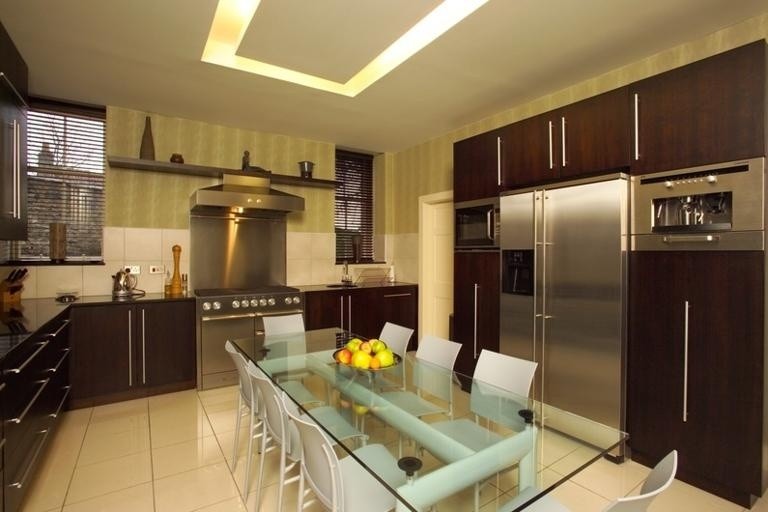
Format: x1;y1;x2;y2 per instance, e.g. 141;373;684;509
497;178;630;435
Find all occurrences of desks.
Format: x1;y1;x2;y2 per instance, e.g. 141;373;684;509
230;326;632;512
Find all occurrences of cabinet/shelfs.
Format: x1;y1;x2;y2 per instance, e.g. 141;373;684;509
451;248;500;394
451;124;504;205
72;301;197;413
631;34;768;176
505;82;631;191
626;250;768;509
290;288;419;352
2;51;28;243
0;308;75;512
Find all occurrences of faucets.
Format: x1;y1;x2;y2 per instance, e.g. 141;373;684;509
343;261;350;284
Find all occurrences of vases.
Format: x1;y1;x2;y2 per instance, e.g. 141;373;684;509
139;115;158;161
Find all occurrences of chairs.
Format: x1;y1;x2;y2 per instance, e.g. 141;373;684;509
281;387;407;512
248;357;372;512
418;347;539;512
324;320;414;432
361;333;464;461
261;312;315;382
605;448;679;512
224;339;323;503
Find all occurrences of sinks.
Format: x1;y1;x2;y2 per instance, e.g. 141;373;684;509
326;285;358;288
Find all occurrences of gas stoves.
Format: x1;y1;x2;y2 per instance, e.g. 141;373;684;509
193;287;307;393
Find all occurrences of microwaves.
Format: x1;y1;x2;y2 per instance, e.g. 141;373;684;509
452;196;500;255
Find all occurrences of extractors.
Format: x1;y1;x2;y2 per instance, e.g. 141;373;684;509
188;171;306;215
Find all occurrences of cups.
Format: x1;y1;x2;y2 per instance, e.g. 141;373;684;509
329;348;405;372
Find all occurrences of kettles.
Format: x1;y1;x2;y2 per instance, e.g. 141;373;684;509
109;268;139;303
